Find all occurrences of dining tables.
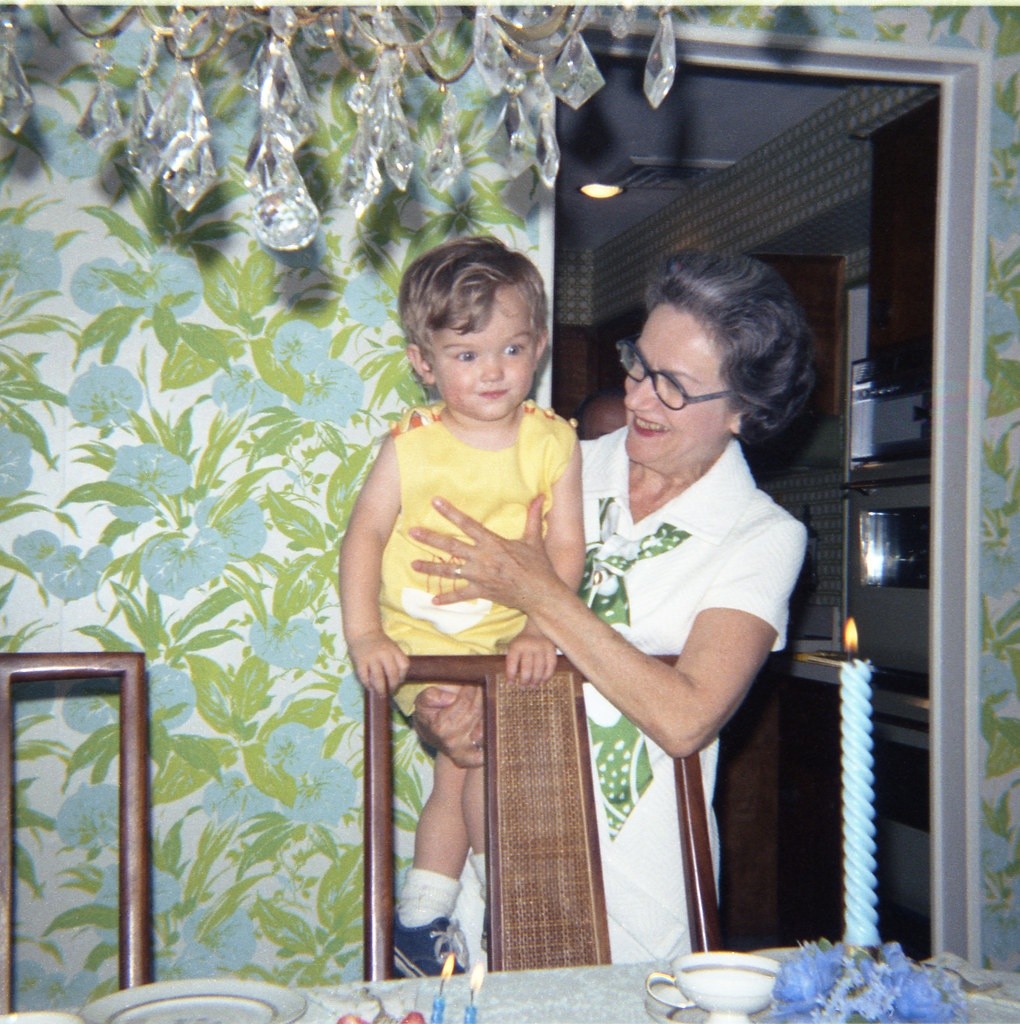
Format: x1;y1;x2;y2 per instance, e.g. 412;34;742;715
2;931;1020;1024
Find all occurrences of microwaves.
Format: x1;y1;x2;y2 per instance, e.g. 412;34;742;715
849;336;938;487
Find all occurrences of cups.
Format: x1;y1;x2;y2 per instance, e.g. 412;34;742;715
643;948;781;1024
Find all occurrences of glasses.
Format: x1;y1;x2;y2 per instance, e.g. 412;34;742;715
617;332;742;408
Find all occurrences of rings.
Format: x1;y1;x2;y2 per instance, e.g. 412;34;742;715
468;733;483;752
455;564;463;579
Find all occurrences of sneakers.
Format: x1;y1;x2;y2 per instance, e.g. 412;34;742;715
391;912;464;979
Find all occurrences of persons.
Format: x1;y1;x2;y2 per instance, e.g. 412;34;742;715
341;239;586;980
414;244;808;974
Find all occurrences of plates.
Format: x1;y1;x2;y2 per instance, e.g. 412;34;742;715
72;978;304;1024
0;1009;84;1024
647;989;788;1024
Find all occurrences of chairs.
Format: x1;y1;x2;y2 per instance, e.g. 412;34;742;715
364;646;727;990
3;649;155;1021
